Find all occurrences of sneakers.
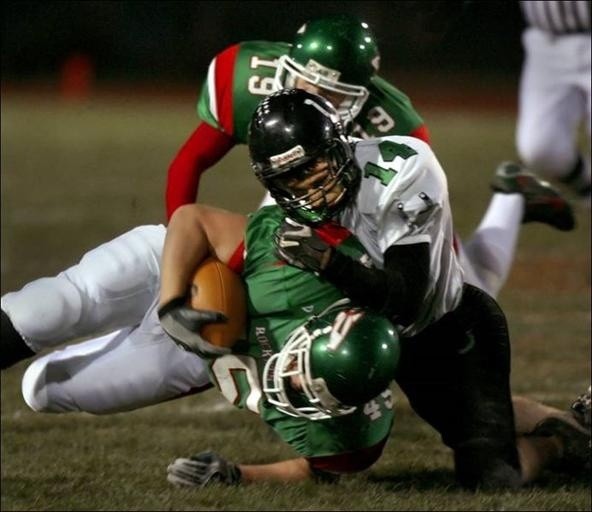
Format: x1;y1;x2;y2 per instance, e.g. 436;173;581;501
489;159;577;234
525;394;591;464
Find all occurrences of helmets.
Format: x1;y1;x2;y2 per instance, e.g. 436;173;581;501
273;15;382;140
262;305;401;422
247;86;363;228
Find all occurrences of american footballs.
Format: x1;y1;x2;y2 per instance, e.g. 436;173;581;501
186;254;251;349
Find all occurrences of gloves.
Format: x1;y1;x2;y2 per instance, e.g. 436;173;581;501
270;218;327;274
163;449;243;489
155;295;234;360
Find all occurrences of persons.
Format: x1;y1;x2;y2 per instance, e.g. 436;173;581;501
165;16;576;306
244;85;592;491
512;1;592;200
1;201;403;494
